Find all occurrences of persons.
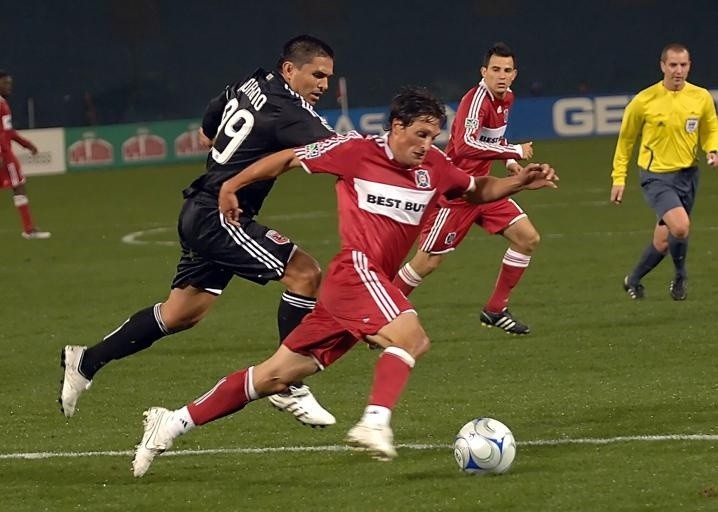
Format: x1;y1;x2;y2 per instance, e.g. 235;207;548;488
130;85;561;480
365;41;540;351
0;74;52;240
610;42;718;300
57;35;348;426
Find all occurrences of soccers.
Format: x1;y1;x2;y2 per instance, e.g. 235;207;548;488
454;417;516;475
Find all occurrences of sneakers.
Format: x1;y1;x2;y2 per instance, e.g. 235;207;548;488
480;307;530;335
59;344;91;418
670;277;687;300
268;385;337;427
343;426;396;461
624;276;644;300
21;227;52;239
132;406;173;477
